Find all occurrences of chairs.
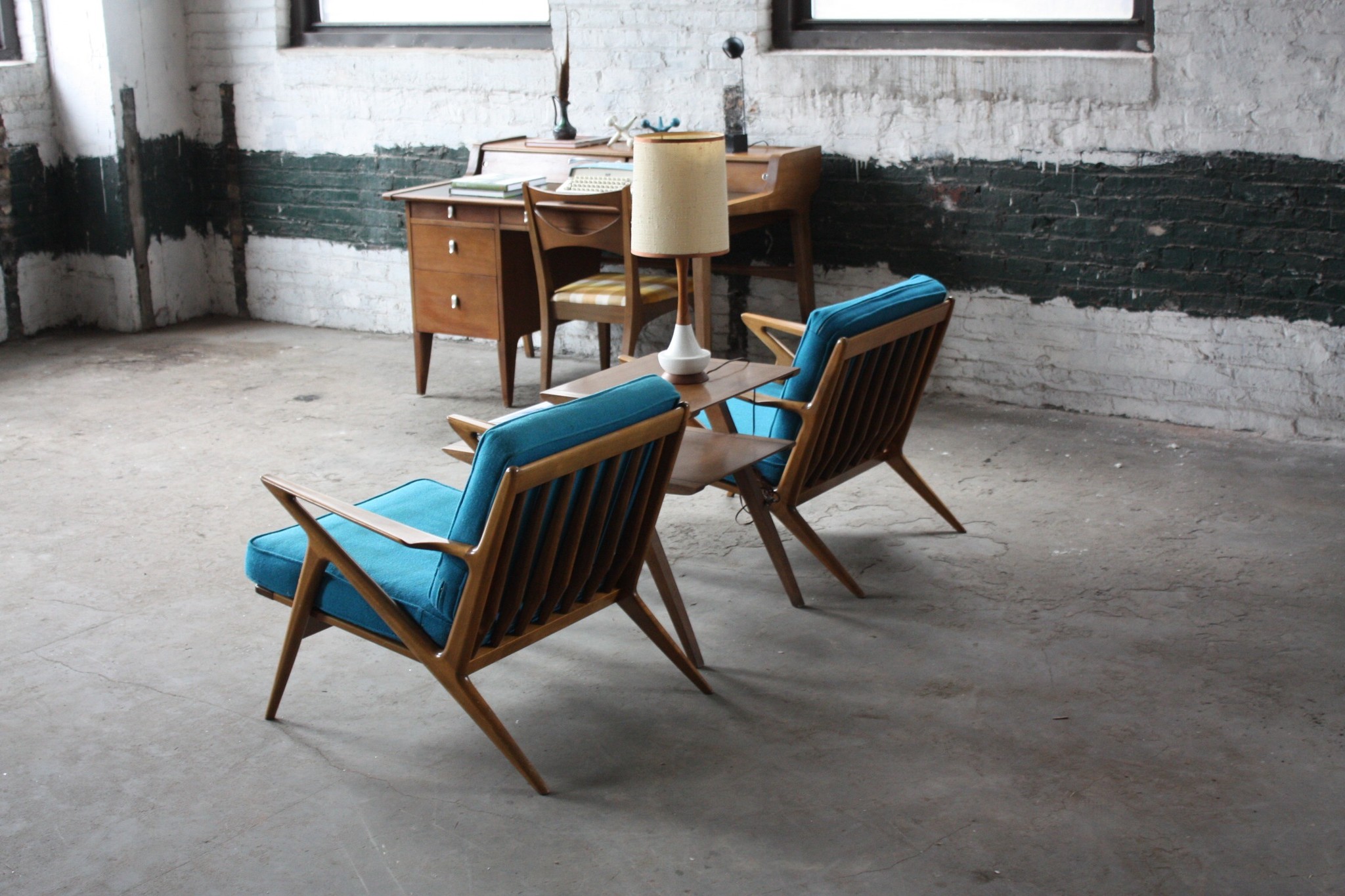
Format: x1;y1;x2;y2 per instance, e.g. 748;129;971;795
522;181;695;391
619;275;970;597
246;374;714;798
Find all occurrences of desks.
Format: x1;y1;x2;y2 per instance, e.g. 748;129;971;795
382;136;822;408
444;352;805;667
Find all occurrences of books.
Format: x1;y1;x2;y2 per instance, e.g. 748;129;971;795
524;135;613;149
448;171;547;199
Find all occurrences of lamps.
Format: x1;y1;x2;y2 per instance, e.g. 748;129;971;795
631;131;730;384
722;37;747;152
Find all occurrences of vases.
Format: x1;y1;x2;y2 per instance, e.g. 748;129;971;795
553;97;576;140
